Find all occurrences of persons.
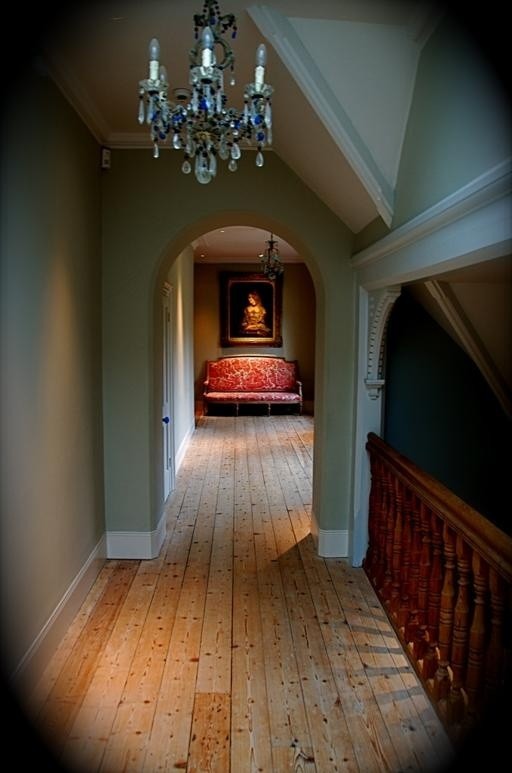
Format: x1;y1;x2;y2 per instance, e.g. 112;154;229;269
240;291;267;334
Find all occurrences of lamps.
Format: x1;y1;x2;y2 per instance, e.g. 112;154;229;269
136;0;275;185
257;234;284;280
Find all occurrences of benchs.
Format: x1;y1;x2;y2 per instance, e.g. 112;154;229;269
202;356;303;417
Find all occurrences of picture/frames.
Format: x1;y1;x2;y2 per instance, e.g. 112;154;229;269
218;271;283;347
